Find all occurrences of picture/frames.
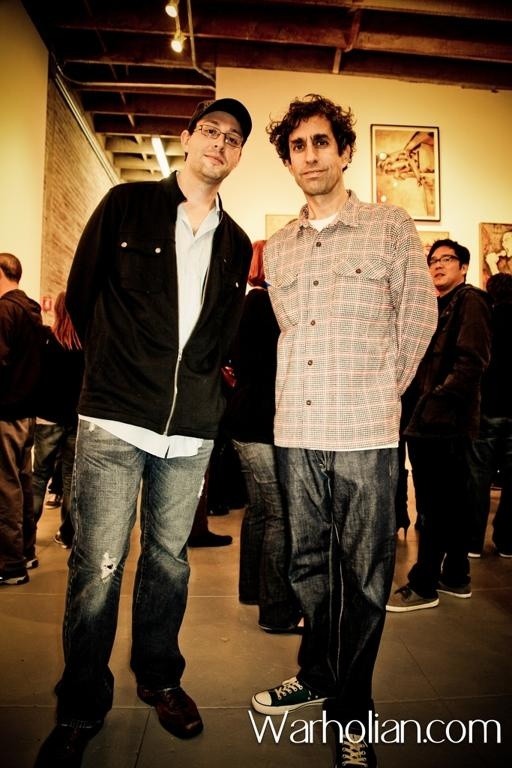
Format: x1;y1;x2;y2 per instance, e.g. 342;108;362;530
477;222;512;290
370;122;444;219
416;229;451;253
262;213;296;236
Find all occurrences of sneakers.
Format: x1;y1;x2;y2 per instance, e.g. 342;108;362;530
385;582;439;612
333;721;378;768
45;495;60;509
260;616;305;634
0;557;39;584
437;579;473;598
252;675;328;715
468;540;512;558
53;530;70;550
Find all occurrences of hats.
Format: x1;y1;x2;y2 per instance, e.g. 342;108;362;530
188;97;252;147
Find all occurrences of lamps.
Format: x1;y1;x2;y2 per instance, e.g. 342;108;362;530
160;1;189;53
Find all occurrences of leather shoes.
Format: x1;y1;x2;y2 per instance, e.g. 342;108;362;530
188;531;232;547
137;682;202;739
34;706;104;768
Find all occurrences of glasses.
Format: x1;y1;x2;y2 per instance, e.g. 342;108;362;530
193;124;243;149
429;255;460;268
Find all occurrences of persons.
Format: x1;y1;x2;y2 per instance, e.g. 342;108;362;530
39;97;257;767
2;236;512;635
251;96;442;767
